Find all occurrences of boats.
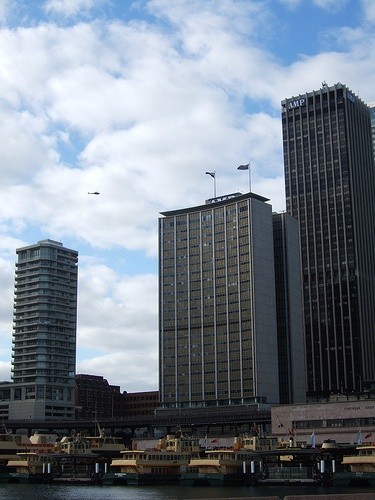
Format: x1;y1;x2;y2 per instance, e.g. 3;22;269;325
107;433;192;486
257;436;329;486
331;443;375;488
177;436;254;487
0;432;125;483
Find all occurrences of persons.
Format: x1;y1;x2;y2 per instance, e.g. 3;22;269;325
69;435;74;444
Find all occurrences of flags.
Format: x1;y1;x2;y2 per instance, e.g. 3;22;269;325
238;162;251;172
205;171;215;178
